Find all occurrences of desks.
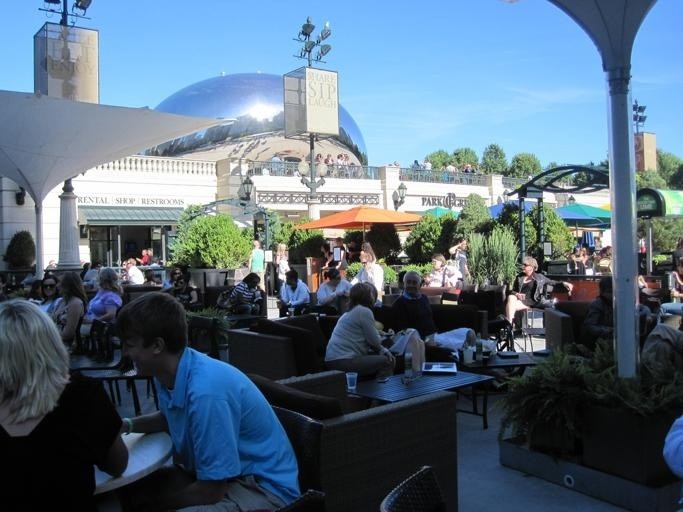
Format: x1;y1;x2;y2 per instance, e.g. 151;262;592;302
450;350;552;416
89;426;176;497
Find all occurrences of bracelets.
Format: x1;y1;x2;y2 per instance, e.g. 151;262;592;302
125;418;132;435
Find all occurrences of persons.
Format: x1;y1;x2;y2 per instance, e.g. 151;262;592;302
367;281;400;336
84;263;101;285
673;238;683;261
163;267;182;291
322;243;333;268
393;270;438;337
351;243;385;304
426;254;462;293
125;258;144;285
322;284;396;375
317;270;353;315
30;281;43;299
159;274;197;304
668;264;683;300
569;243;614;270
324;237;347;271
122;260;128;276
143;270;159;285
0;271;22;297
575;277;617;345
118;292;301;511
147;249;158;265
137;249;147;265
79;262;89;280
0;299;128;512
275;243;290;294
81;268;121;340
45;272;89;352
507;258;573;329
348;240;360;264
448;237;471;283
249;241;266;290
36;274;59;314
280;270;311;317
230;273;261;314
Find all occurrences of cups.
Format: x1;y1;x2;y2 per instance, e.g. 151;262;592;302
345;372;358;390
288;308;295;317
463;348;474;365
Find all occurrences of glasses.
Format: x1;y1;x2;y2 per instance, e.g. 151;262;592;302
175;280;183;285
252;281;257;284
122;263;126;265
522;263;531;266
42;283;55;289
173;272;180;275
126;263;131;264
95;265;100;268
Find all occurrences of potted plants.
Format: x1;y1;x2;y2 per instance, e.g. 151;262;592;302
169;214;254;293
577;338;682;486
185;306;230;352
488;343;619;460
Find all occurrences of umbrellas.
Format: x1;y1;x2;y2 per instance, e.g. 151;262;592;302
425;206;458;220
291;203;424;242
551;207;596;220
563;203;612;242
486;200;540;219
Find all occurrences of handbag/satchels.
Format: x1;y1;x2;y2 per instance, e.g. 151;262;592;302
176;294;189;304
217;286;242;310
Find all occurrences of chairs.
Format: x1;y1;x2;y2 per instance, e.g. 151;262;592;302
80;350;142;418
67;324;129;406
277;488;325;511
64;318;115;406
378;463;447;512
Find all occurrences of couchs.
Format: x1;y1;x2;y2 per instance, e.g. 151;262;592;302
548;280;600;304
319;303;480;378
226;313;328;383
543;300;601;357
310;292;350;316
125;284;162;305
204;284;268;316
244;369;459;511
